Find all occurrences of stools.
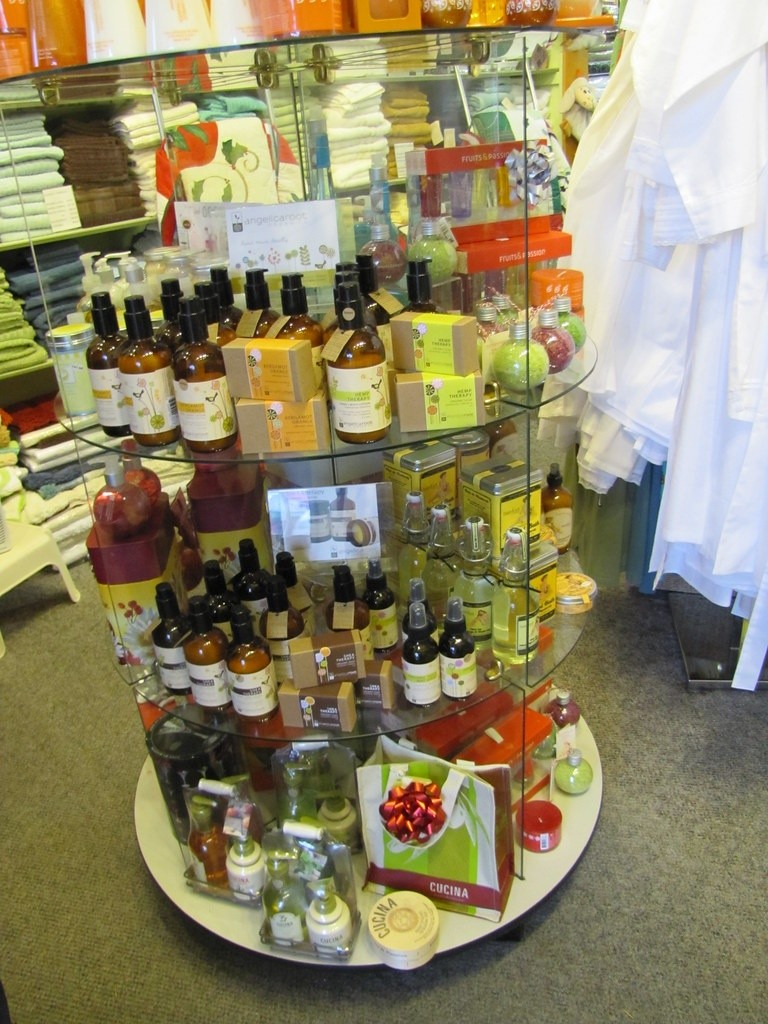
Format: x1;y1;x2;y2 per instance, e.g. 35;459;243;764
0;521;82;661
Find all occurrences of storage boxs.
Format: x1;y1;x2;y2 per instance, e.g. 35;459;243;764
234;388;334;456
394;370;487;434
386;311;480;378
221;338;318;402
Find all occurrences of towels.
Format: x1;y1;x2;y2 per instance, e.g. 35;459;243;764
0;32;573;576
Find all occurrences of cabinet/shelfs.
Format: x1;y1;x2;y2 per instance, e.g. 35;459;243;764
0;23;626;533
49;313;596;965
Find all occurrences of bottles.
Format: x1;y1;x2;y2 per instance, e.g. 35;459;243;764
47;99;592;960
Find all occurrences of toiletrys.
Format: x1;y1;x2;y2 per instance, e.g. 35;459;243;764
42;144;599;971
